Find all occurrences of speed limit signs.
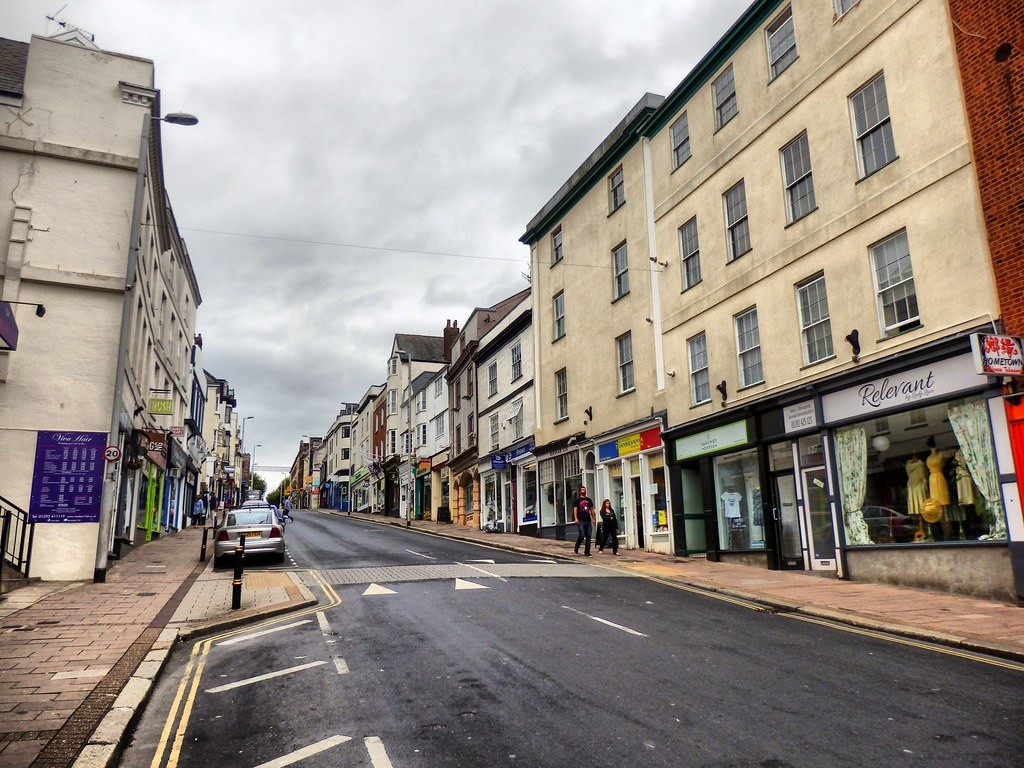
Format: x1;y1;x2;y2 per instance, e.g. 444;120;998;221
104;446;121;461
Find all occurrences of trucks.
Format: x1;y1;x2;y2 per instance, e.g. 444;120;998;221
244;490;264;501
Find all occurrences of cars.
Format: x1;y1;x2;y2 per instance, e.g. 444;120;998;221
214;500;288;566
822;504;918;543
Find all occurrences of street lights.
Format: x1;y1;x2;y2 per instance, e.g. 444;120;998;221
239;416;255;507
395;349;412;527
94;112;199;583
341;401;354;515
251;445;262;491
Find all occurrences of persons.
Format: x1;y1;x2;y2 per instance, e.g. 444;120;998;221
599;499;621;557
955;449;974;505
574;486;596;558
209;492;218;527
906;457;927;514
927;448;951;505
282;495;294;525
192;494;203;529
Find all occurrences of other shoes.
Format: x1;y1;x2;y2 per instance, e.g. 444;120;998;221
599;550;603;554
292;518;293;522
613;552;621;556
585;553;593;558
574;545;578;554
